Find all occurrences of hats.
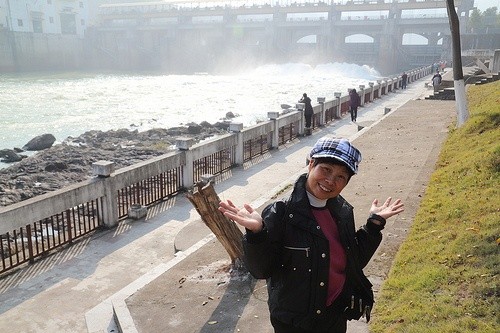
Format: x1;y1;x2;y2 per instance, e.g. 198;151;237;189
307;134;362;176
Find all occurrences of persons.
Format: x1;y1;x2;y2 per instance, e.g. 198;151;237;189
298;93;313;129
349;89;359;122
402;72;407;89
217;138;404;332
431;60;447;87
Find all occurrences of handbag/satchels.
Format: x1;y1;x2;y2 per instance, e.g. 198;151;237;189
343;270;375;323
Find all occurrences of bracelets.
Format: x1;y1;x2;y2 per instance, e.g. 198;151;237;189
368;213;386;226
